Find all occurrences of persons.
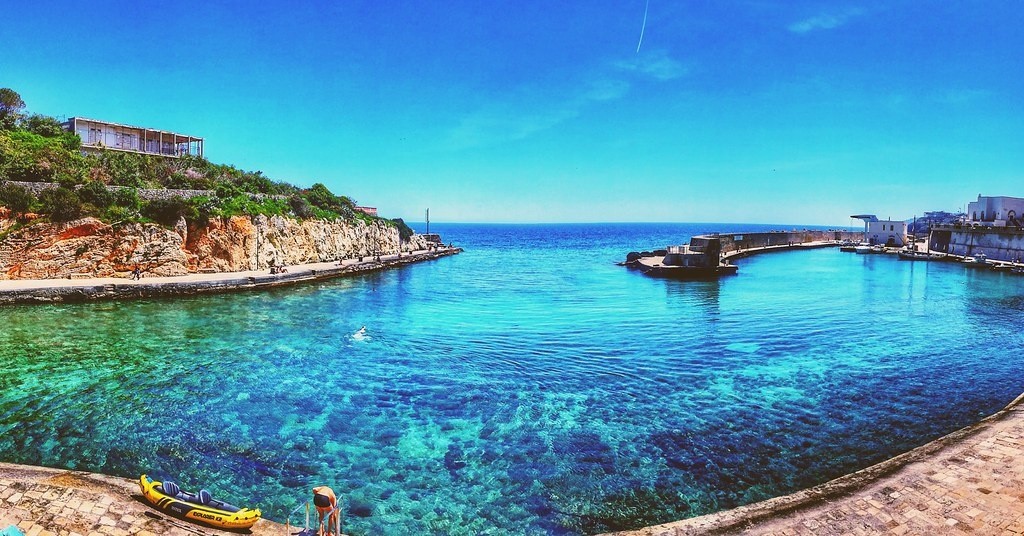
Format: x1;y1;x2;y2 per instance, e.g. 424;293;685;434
434;242;438;252
398;254;402;260
279;265;287;273
788;240;791;246
132;262;140;280
448;243;452;248
313;485;338;536
339;259;342;265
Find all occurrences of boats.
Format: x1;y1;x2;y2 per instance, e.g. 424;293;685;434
854;242;872;254
139;473;262;530
871;244;884;254
960;260;992;267
897;216;949;262
